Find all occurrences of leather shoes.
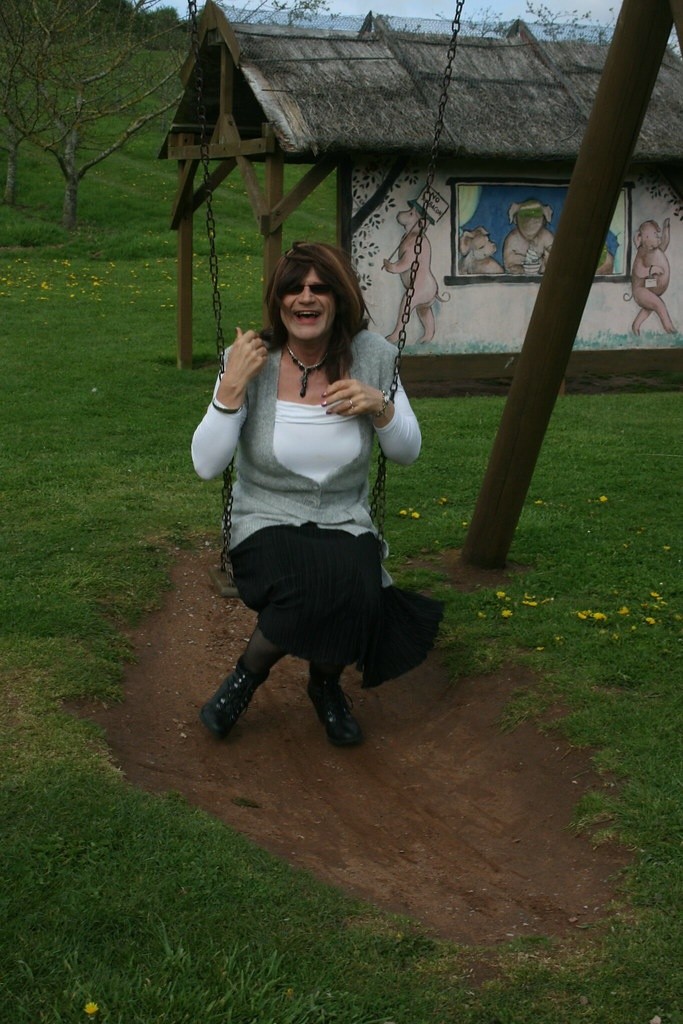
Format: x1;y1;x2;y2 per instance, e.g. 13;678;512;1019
307;661;362;745
200;654;270;742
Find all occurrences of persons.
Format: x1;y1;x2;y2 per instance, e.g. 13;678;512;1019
191;244;421;748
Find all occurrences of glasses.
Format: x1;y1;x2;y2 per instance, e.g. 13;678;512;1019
277;284;335;294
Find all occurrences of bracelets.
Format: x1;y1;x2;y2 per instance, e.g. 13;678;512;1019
376;390;389;417
211;402;242;414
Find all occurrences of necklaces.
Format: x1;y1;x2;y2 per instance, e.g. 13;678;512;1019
286;343;327;398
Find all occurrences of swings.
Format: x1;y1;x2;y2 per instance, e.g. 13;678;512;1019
183;0;473;610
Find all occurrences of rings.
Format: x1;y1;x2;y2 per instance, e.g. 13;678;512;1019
349;400;353;410
